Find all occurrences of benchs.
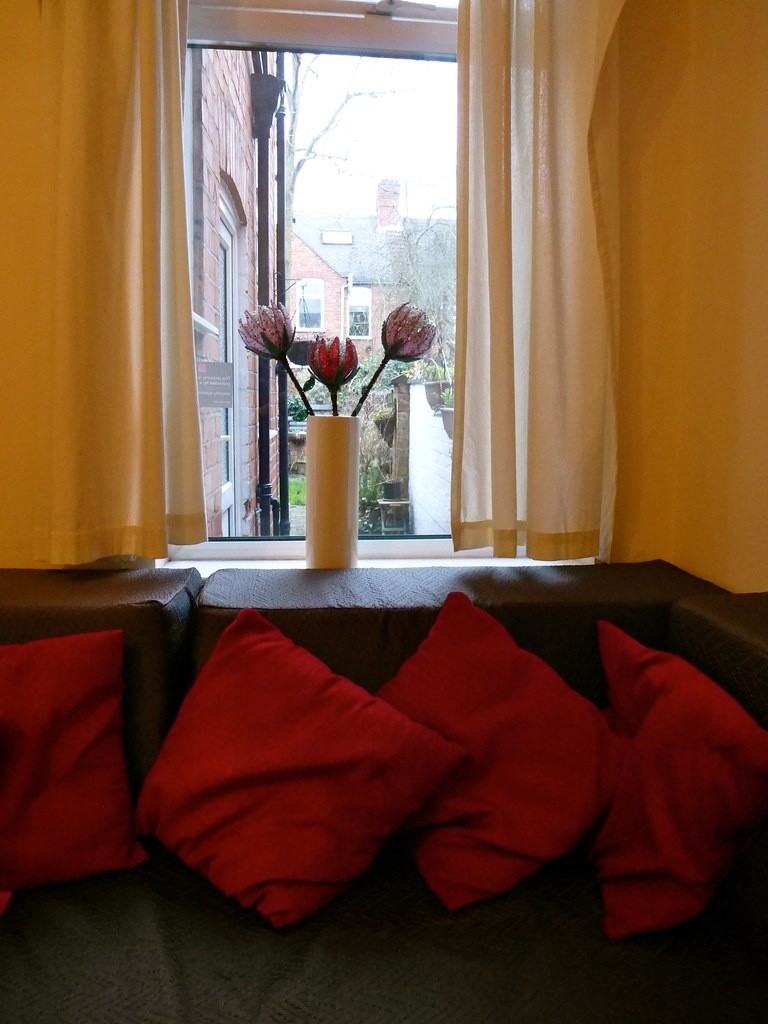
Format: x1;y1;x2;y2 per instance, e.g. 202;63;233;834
0;558;768;1024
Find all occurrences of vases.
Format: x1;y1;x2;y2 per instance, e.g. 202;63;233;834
305;415;361;568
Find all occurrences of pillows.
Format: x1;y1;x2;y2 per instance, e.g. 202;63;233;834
133;607;466;931
0;628;151;888
592;619;768;942
375;590;626;912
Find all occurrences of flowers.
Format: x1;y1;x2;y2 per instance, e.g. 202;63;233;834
239;296;438;413
